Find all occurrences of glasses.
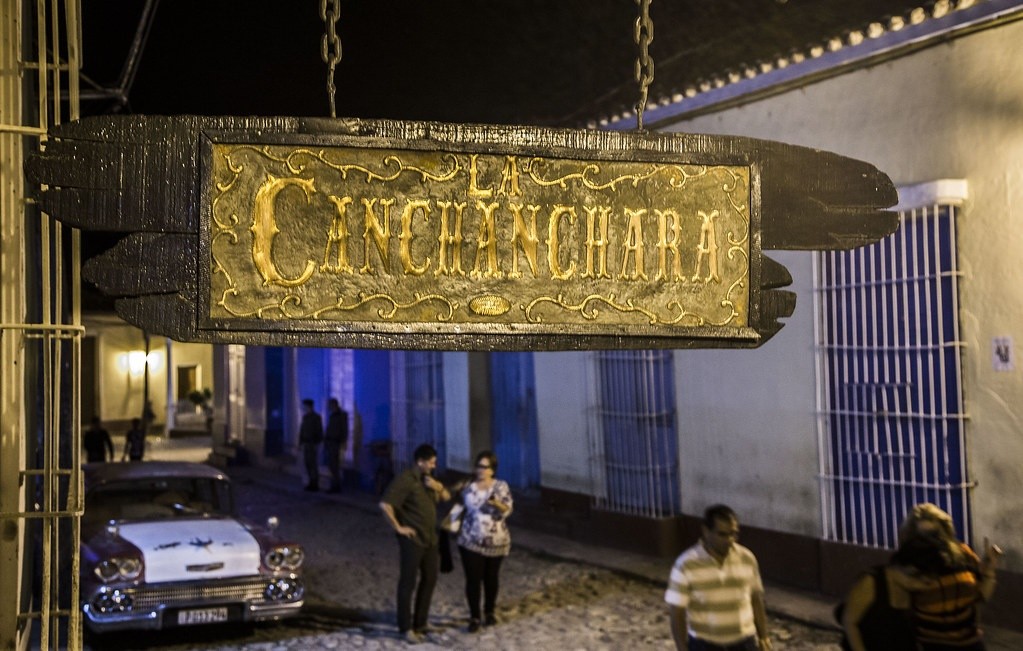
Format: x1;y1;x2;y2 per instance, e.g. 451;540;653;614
476;462;492;470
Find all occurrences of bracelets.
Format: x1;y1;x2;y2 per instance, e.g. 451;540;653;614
439;488;444;493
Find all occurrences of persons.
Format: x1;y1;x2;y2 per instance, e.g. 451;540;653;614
449;451;513;632
298;399;323;491
84;417;113;463
378;445;451;645
325;399;348;493
122;419;144;461
843;503;1003;651
665;504;773;651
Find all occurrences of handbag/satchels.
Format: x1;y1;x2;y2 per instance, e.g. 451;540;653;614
439;478;469;532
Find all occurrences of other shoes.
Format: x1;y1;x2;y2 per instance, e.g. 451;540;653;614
468;616;480;634
485;612;497;625
417;623;446;635
405;630;419;644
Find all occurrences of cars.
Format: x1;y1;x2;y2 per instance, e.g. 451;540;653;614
25;461;309;636
